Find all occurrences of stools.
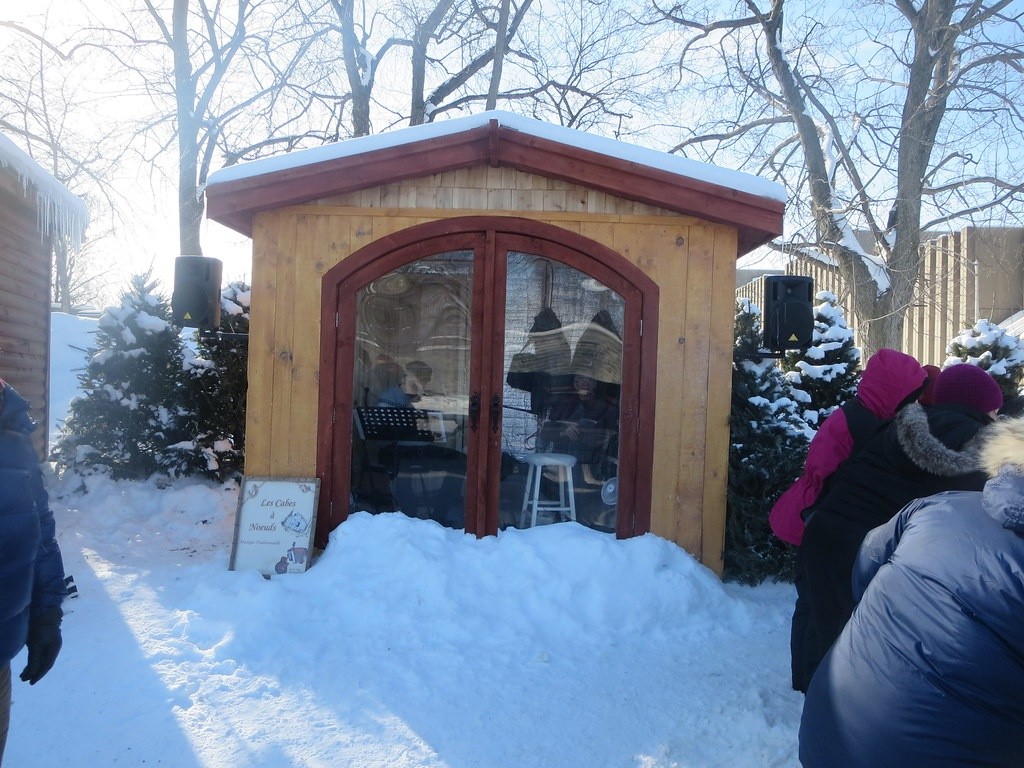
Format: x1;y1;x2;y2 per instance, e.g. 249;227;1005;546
521;452;577;530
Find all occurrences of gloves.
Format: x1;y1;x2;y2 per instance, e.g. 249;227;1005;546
20;604;63;686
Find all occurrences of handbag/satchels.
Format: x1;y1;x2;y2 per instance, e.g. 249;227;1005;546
506;353;536;391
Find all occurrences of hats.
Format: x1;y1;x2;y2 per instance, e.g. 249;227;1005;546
918;364;1003;413
375;363;406;382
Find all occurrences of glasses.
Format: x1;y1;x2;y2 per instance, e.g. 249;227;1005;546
575;375;594;382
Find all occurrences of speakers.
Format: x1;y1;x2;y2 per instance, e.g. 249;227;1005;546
171;256;222;329
763;274;814;351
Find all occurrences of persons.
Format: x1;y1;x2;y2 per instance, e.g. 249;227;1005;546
798;415;1024;767
0;376;65;768
375;354;466;476
768;349;930;696
540;367;618;488
803;364;1003;648
